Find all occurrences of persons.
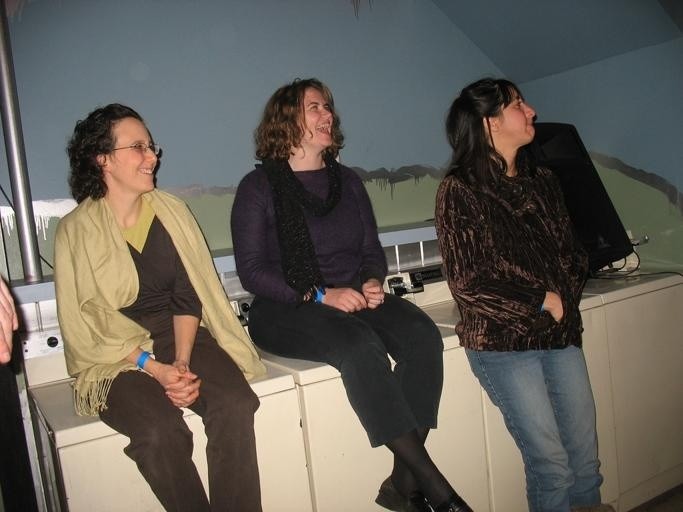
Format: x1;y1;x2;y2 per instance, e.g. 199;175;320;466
429;75;618;512
49;102;272;512
0;274;21;370
225;76;477;512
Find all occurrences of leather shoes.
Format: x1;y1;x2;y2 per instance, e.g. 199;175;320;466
425;492;474;512
374;473;433;512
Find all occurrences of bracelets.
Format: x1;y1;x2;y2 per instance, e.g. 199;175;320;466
135;351;151;369
539;304;546;313
314;285;324;304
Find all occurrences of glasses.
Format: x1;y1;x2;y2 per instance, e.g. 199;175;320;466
111;142;161;156
